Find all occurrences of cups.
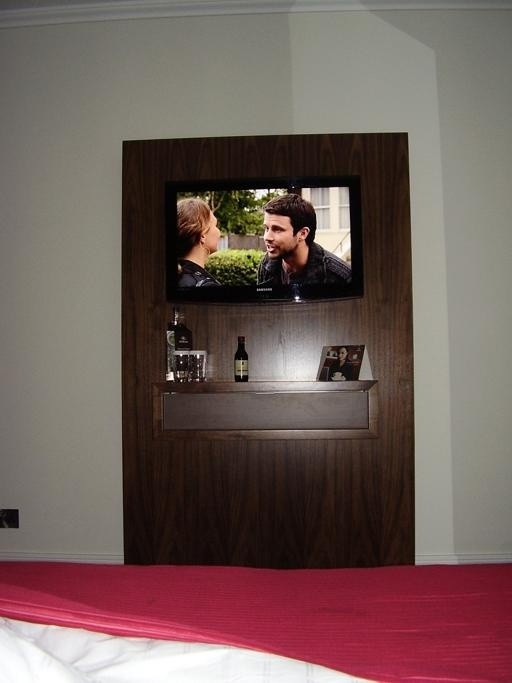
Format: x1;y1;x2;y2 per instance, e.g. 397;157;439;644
173;354;205;383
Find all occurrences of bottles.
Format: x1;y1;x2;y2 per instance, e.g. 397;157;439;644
234;336;249;382
174;311;193;350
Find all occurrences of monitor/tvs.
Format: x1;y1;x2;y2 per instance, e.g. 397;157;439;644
163;173;364;305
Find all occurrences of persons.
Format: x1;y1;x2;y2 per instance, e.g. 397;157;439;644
257;193;352;286
177;198;225;288
328;346;354;381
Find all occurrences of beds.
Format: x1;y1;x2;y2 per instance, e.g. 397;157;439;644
1;559;511;681
